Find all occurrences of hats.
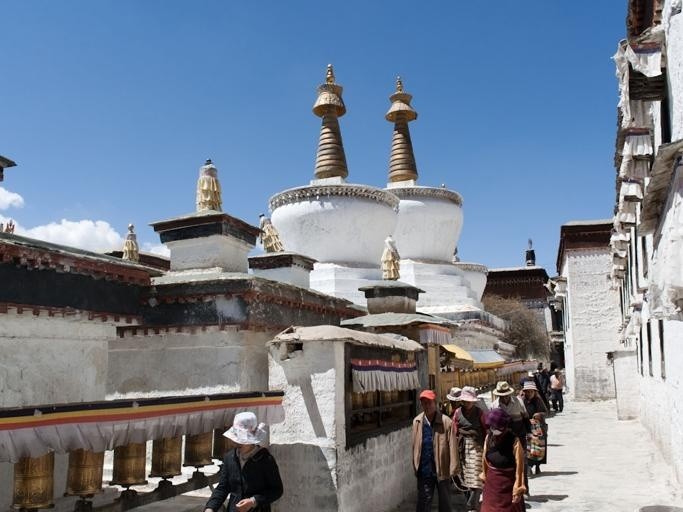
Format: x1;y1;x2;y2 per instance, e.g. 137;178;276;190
492;380;515;397
418;389;436;401
522;380;539;392
553;368;561;374
484;408;513;429
446;386;462;402
460;385;481;402
221;411;267;446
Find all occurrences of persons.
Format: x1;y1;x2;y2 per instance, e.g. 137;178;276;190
409;360;569;512
201;410;284;512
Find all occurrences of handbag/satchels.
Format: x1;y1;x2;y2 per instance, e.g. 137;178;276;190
523;418;547;462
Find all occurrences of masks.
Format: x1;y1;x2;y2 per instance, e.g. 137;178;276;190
489;426;503;436
